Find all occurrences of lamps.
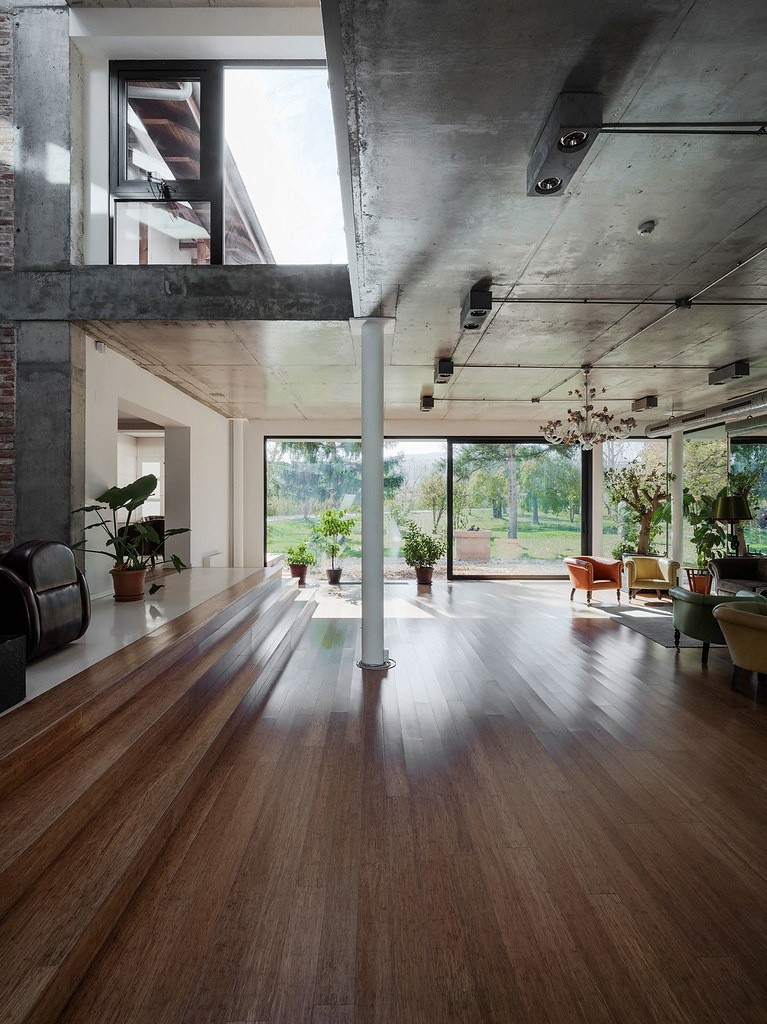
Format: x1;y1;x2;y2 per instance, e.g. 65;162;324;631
713;496;753;557
538;365;638;451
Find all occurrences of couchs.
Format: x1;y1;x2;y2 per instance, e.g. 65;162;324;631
565;556;624;608
668;587;767;657
708;557;767;596
0;539;92;661
712;602;767;689
624;557;680;603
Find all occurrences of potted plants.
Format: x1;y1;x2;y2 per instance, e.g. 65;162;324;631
715;463;764;557
604;459;676;561
69;474;192;602
683;487;728;594
309;508;357;584
284;537;318;585
401;518;449;584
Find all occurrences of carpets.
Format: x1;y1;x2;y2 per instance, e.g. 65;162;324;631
610;617;727;648
